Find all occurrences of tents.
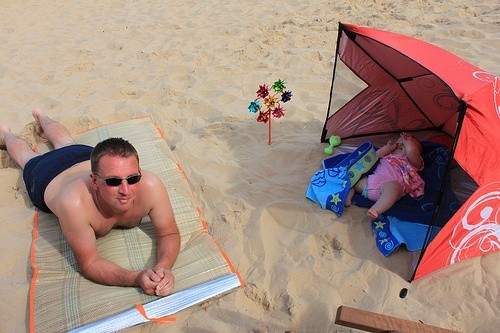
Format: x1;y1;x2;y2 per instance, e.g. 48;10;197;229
321;22;500;282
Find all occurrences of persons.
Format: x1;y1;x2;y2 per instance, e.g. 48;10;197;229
345;133;425;220
0;107;181;296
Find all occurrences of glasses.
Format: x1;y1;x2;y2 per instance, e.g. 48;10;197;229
94;170;142;186
